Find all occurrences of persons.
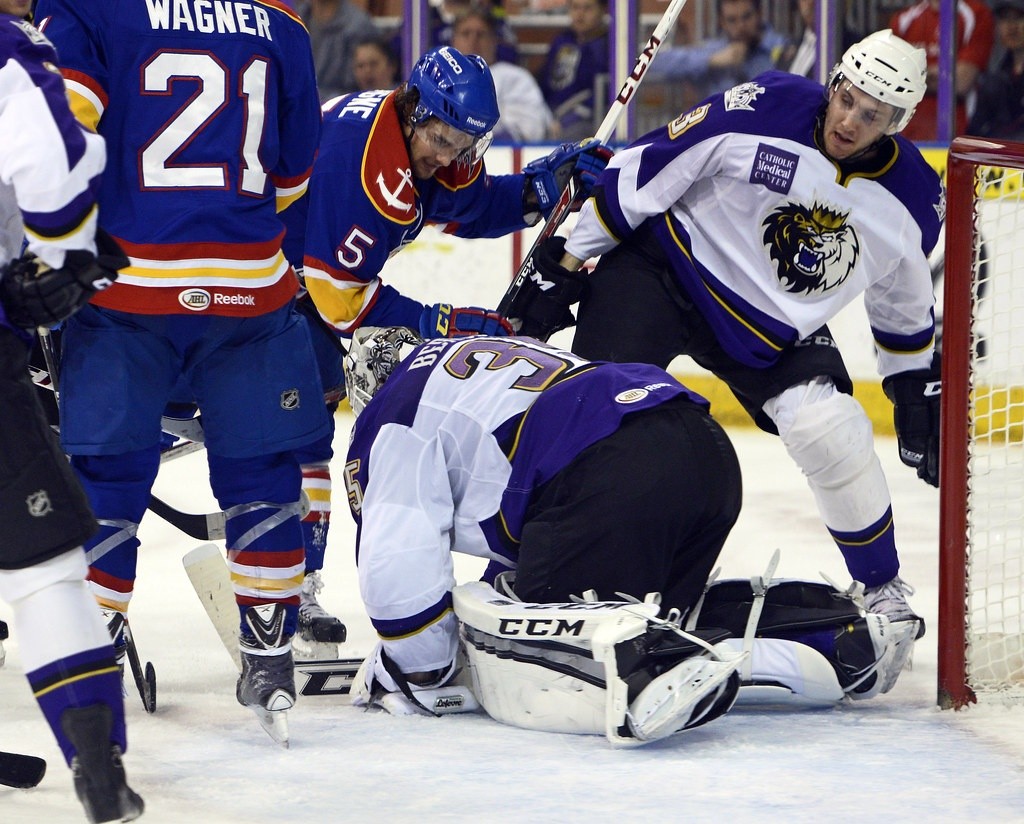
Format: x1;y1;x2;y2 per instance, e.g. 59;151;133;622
280;0;1024;141
273;47;614;663
344;324;918;749
33;0;324;746
0;0;146;824
508;30;940;635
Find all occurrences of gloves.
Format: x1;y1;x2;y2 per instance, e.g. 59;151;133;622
419;303;515;339
510;236;588;343
1;225;131;328
350;642;479;716
881;367;939;489
523;135;613;221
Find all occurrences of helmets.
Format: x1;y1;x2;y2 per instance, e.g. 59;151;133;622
345;326;426;418
405;45;501;139
828;28;928;136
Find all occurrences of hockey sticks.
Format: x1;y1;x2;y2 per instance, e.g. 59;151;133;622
493;0;687;319
0;751;48;790
159;386;350;466
178;543;365;699
51;425;313;542
26;364;206;444
20;259;158;714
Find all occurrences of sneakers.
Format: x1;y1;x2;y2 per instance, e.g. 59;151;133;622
862;573;926;671
291;571;347;658
236;603;297;750
626;651;751;742
98;607;129;698
834;613;920;700
62;701;144;824
